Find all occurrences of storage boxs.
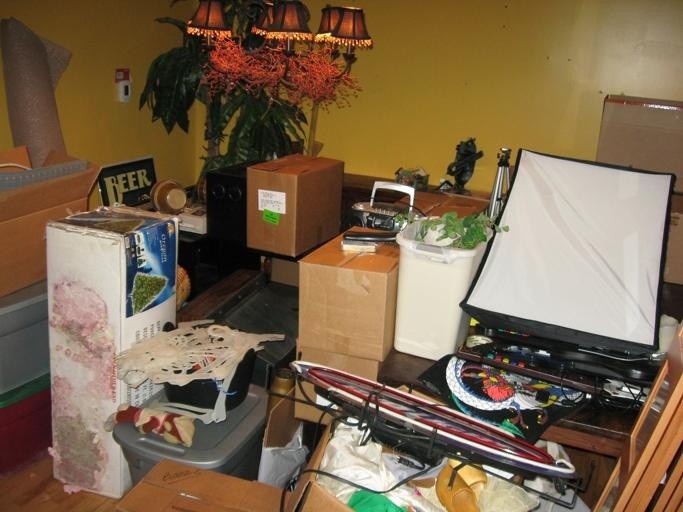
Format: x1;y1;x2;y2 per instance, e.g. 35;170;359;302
1;277;52;396
391;215;493;362
110;458;293;509
297;191;491;362
592;93;678;194
663;193;678;287
256;385;313;492
2;143;104;301
248;154;345;257
111;381;270;487
288;418;592;510
296;338;379;426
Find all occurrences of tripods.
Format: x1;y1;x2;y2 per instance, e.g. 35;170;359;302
488;148;511;219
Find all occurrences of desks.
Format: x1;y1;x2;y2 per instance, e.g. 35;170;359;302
376;345;631;459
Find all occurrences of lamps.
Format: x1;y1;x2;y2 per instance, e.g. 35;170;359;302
186;1;378;189
